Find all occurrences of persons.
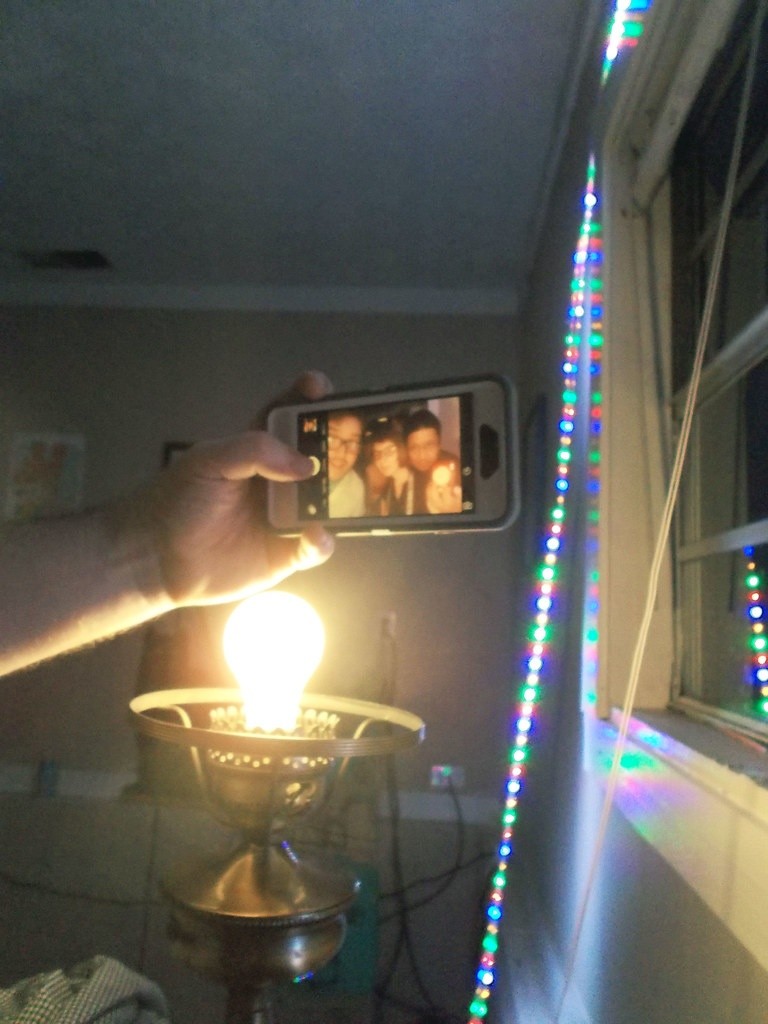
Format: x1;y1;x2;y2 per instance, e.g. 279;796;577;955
328;407;462;517
0;366;337;679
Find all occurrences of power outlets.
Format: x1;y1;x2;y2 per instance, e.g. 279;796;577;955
430;764;465;789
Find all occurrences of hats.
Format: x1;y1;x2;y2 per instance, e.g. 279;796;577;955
365;415;406;442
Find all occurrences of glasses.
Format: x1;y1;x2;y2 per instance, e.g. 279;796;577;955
327;434;361;455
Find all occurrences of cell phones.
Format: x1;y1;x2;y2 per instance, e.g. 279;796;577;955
262;371;522;535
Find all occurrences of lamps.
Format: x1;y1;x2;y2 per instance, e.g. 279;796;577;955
126;587;425;1024
